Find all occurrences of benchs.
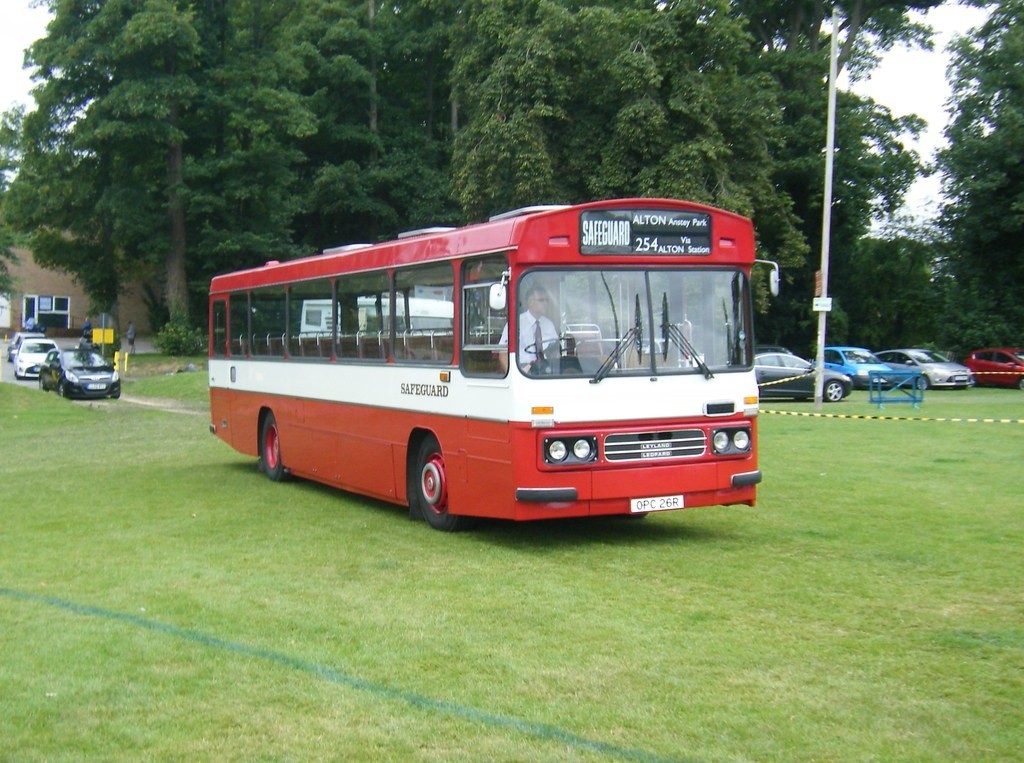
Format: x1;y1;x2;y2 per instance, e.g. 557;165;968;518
230;324;603;373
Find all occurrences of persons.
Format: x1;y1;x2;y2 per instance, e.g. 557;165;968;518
25;314;35;332
126;320;136;354
82;316;92;343
499;285;560;376
36;320;47;333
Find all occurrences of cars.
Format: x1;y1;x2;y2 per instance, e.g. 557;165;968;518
8;332;45;362
813;347;895;390
963;348;1024;390
39;348;121;399
11;339;59;379
874;349;977;391
753;352;853;403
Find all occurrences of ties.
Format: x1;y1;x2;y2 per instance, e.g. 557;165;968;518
535;321;544;360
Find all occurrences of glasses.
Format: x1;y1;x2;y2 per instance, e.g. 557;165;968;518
534;298;549;301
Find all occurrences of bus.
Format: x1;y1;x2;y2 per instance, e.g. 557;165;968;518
208;198;780;533
